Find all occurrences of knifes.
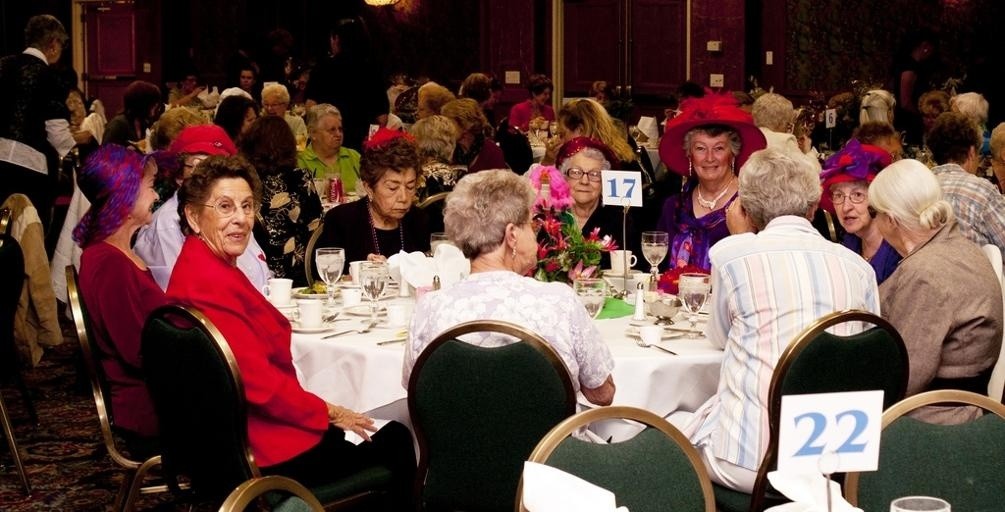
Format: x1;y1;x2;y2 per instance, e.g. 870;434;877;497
680;308;708;316
659;327;706;342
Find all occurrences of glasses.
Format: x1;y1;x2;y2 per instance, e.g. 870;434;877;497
562;167;602;184
195;195;264;219
829;192;866;206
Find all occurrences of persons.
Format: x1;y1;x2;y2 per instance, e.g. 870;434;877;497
1;15;1005;512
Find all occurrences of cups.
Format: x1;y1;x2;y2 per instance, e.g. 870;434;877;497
261;277;294;306
549;121;559;138
649;137;658;148
917;150;928;164
609;249;638;274
574;276;606;318
313;177;324;199
630;128;640;141
291;299;321;327
539;121;548;130
430;232;454;255
354;178;367;198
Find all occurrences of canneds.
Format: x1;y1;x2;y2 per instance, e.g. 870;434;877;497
328;177;343;204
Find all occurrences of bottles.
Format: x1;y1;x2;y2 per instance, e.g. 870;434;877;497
634;281;647;320
433;276;441;290
400;275;411;299
648;276;658;291
541;184;551;209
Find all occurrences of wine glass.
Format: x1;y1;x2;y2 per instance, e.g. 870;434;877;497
677;272;712;340
313;246;391;325
641;230;669;281
647;296;683;326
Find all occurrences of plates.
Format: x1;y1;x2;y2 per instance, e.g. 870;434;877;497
288;322;337;335
360;288;398;300
291;287;340;299
646;146;659;150
603;269;634;278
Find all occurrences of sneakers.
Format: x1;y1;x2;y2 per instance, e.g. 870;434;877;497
39;339;74;356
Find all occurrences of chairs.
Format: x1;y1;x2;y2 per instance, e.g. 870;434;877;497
0;147;1005;511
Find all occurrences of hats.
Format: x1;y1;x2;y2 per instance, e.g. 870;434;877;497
819;137;893;215
553;137;613;166
659;88;766;175
71;145;154;245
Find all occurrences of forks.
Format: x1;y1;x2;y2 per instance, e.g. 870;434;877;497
633;335;677;357
322;322;377;339
